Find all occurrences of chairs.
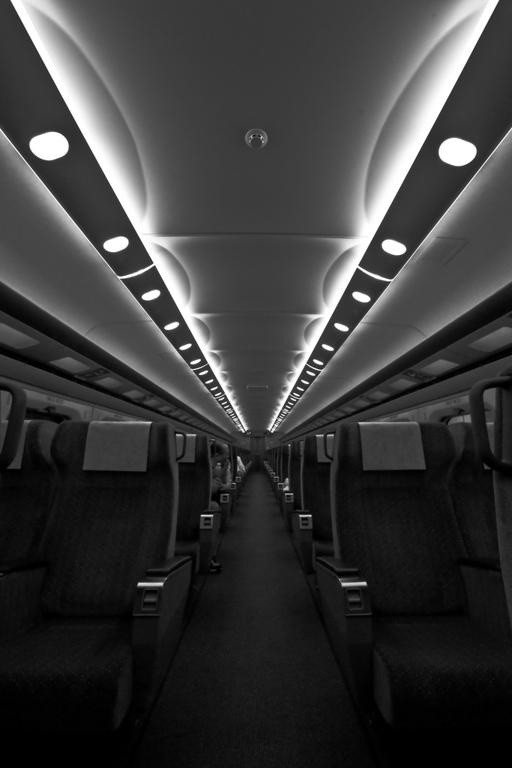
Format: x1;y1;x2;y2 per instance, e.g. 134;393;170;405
313;420;511;763
258;431;337;574
447;418;511;647
175;431;254;574
2;419;194;767
469;366;512;630
1;420;56;642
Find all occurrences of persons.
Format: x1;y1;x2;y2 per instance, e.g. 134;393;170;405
209;441;246;573
